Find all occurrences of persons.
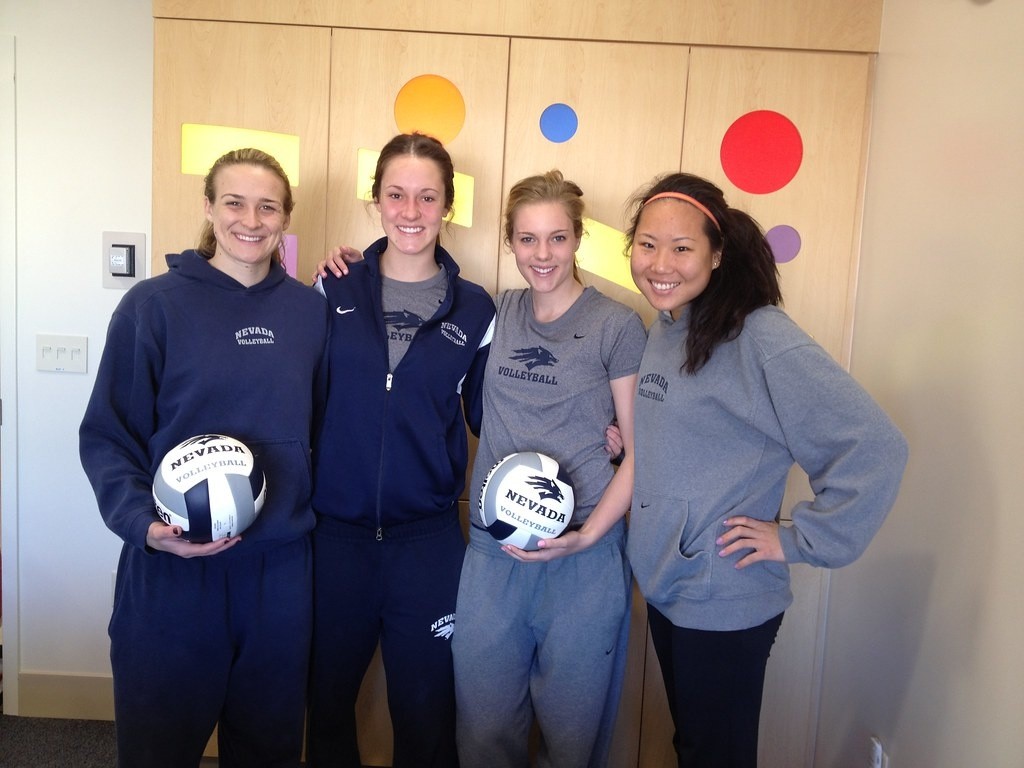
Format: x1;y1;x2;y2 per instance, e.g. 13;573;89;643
79;148;326;768
627;172;910;768
311;171;646;768
310;131;623;768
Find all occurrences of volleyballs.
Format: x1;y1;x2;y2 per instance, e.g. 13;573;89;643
478;451;577;553
152;433;267;545
